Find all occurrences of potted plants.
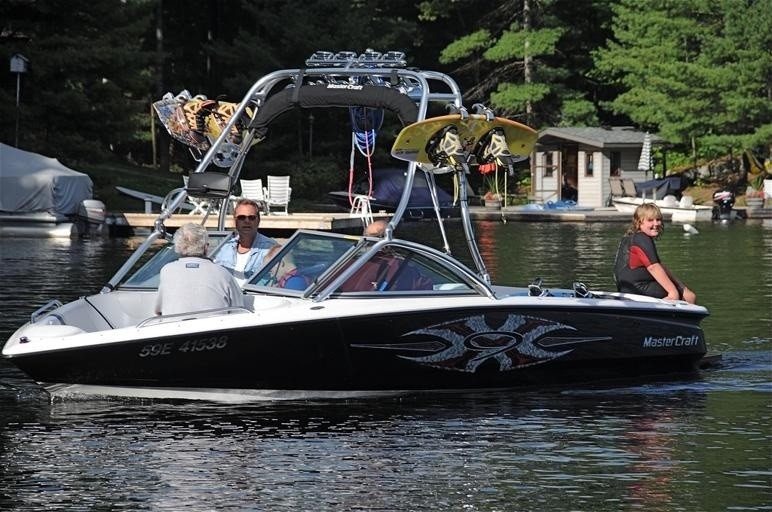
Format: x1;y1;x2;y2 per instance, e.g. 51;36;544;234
481;173;511;209
744;176;765;209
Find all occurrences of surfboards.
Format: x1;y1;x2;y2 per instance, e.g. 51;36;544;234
153;98;266;153
391;113;540;167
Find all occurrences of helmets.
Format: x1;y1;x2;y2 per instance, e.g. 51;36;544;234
362;218;389;238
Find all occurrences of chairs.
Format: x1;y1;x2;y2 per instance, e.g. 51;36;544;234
238;174;292;216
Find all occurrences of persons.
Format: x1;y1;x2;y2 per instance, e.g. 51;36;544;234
328;221;435;293
614;202;698;306
156;199;311;315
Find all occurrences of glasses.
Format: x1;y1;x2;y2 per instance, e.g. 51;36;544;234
234;213;260;222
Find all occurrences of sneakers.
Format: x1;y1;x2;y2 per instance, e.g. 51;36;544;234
573;281;600;298
528;278;556;297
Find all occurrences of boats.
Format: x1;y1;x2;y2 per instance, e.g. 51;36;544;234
611;187;713;222
1;48;711;402
0;142;108;224
